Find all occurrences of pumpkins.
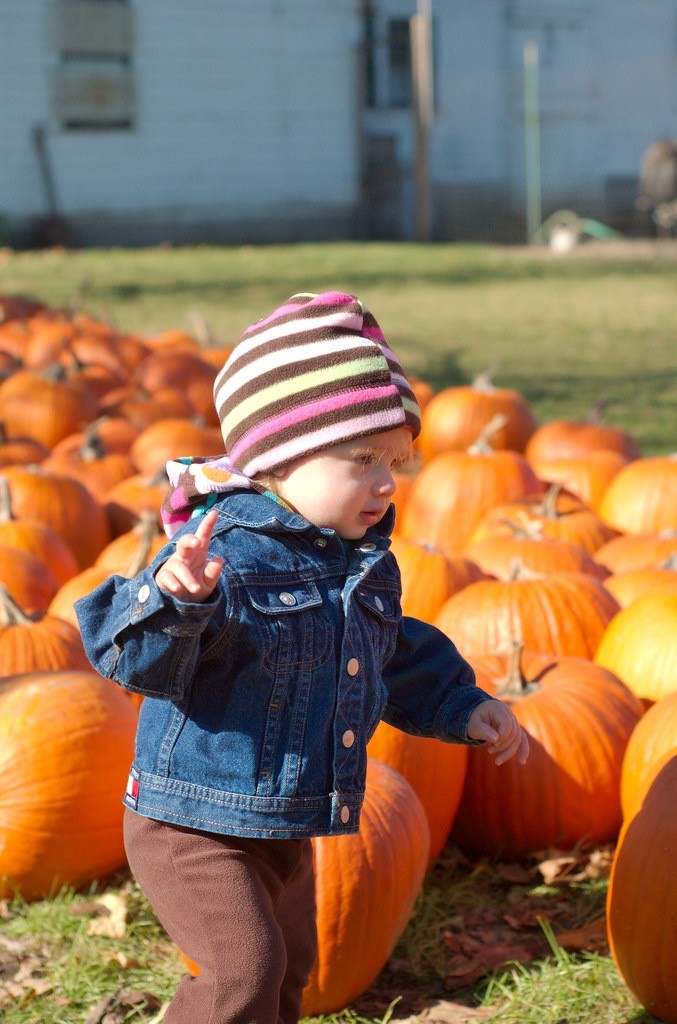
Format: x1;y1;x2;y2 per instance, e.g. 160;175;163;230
0;292;677;1024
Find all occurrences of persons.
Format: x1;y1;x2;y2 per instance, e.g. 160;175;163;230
71;289;531;1024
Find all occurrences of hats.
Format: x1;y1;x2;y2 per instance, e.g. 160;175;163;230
212;292;422;478
